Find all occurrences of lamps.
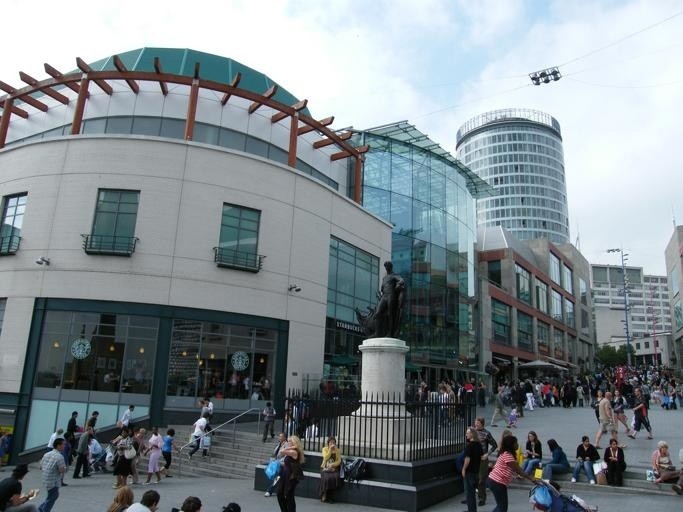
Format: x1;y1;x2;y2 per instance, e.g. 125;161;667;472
288;283;301;292
35;256;50;265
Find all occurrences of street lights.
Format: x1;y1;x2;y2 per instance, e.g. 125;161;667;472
646;279;659;368
606;248;630;365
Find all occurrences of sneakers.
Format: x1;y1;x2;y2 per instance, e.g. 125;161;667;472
112;479;161;489
264;492;271;497
321;496;330;503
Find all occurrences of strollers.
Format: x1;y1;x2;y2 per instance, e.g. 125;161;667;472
525;477;596;512
89;443;112;473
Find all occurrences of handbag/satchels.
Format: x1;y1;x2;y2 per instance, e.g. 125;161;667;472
265;457;281;481
288;448;303;481
189;429;211;450
124;437;137;460
339;462;346;479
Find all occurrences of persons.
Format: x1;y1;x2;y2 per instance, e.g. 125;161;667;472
571;435;600;484
407;378;487;426
491;363;683;433
604;439;627;487
369;261;405;338
319;376;362;415
540;439;570;481
265;433;304;512
651;441;683;495
227;372;271;400
283;394;312;439
1;399;240;512
594;392;621;449
460;418;552;512
628;388;654;440
319;437;341;503
262;402;276;442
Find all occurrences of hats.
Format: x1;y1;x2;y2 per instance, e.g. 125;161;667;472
12;463;29;472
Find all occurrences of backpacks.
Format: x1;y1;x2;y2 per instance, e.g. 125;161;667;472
344;457;367;488
